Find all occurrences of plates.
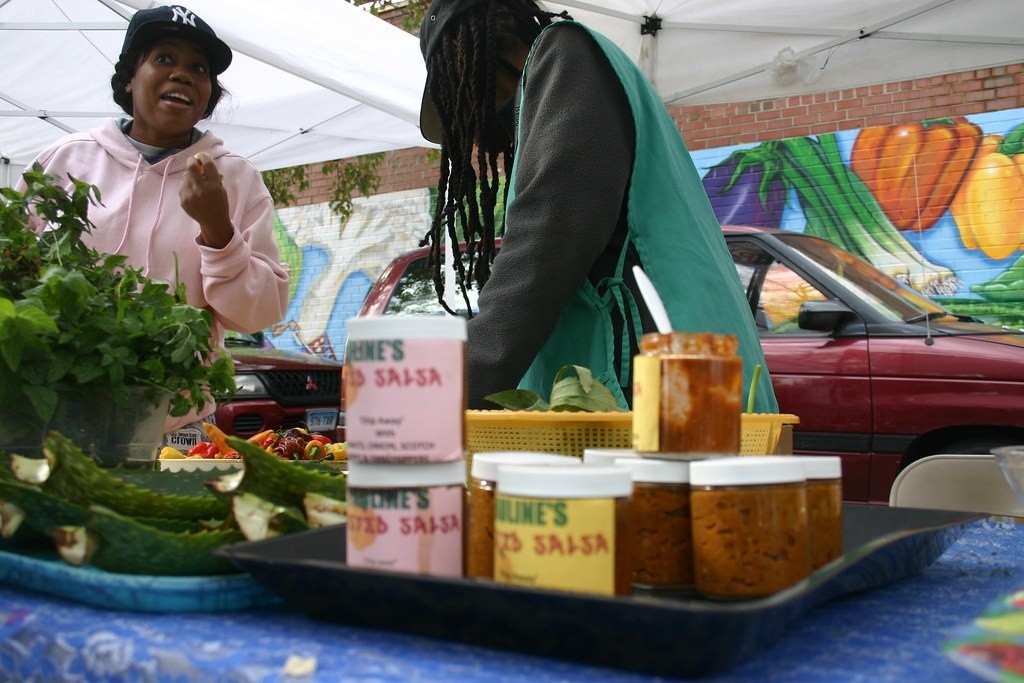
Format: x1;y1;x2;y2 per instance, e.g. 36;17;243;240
0;550;268;613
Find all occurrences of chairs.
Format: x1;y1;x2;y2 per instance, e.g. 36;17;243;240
890;451;1023;515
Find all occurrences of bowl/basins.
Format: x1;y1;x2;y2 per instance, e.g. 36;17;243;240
990;445;1024;503
157;457;293;472
0;385;170;470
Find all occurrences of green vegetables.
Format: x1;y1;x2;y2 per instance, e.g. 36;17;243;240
0;160;241;471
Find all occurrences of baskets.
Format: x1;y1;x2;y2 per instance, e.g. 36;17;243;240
464;409;801;487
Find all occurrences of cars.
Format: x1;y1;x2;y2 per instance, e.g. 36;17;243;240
341;228;1023;505
164;332;343;460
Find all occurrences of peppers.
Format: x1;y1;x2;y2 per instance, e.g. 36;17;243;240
156;423;344;462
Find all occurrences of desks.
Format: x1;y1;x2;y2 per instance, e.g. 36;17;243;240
0;503;1024;681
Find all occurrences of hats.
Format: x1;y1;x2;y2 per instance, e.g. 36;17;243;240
419;0;535;144
120;5;232;79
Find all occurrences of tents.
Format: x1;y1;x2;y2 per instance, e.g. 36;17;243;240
0;0;1023;170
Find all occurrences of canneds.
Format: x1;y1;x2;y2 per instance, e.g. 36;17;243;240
472;331;842;602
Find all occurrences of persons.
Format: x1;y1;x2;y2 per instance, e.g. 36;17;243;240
8;6;291;460
420;0;781;416
